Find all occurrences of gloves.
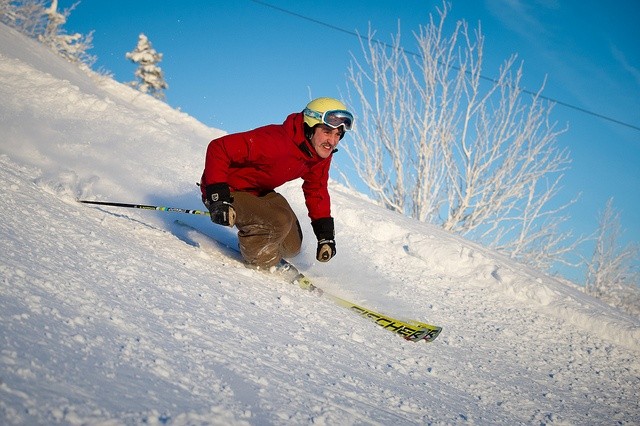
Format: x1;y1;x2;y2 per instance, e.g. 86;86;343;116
205;182;237;226
311;217;336;262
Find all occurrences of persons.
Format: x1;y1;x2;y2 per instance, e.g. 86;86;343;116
196;98;355;282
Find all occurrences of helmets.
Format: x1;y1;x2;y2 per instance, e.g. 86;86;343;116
304;98;347;138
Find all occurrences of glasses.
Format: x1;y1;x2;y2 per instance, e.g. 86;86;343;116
304;106;354;132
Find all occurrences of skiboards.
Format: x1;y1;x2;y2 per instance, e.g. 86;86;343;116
175;218;443;343
